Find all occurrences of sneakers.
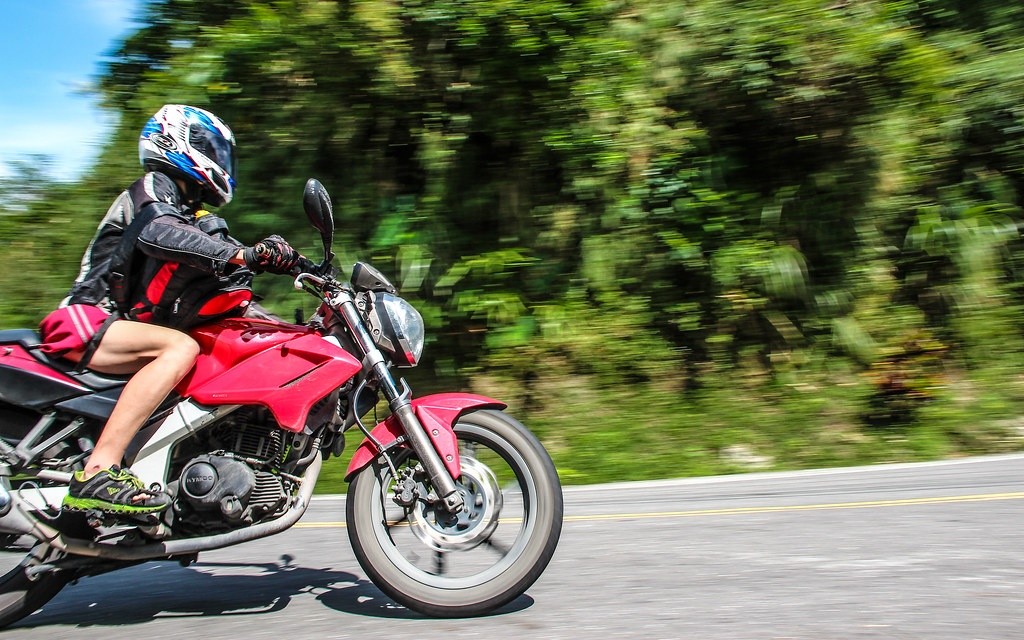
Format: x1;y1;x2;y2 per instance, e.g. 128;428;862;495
61;463;173;515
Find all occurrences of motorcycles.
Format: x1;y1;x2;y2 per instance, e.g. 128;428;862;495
0;177;564;629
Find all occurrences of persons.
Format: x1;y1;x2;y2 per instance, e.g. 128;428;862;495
40;104;321;515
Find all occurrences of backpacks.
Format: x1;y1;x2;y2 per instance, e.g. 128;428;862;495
73;202;254;374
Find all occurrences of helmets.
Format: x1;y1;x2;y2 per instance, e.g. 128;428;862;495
138;105;237;207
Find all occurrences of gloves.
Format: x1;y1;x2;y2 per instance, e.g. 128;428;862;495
243;234;306;277
